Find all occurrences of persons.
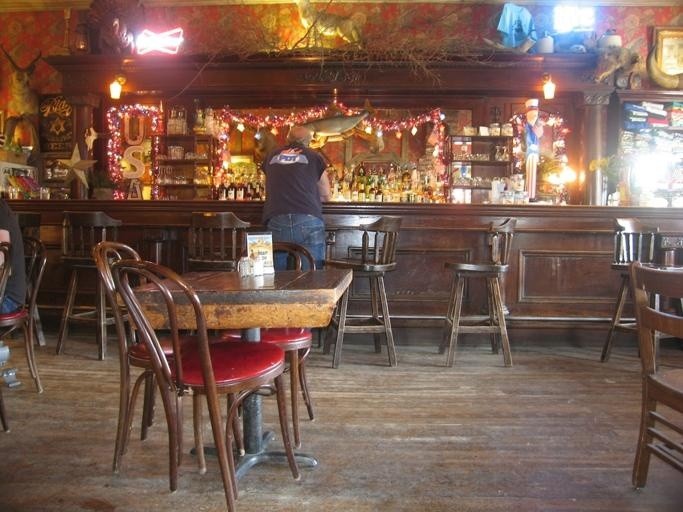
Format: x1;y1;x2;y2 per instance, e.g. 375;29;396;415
0;194;27;317
261;139;330;271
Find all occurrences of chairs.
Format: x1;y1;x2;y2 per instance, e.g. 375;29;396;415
2;232;47;391
227;242;317;447
112;259;300;511
630;261;683;488
92;241;245;493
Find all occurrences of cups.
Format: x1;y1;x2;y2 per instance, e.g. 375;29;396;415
186;153;208;159
167;145;184;160
174;167;209;185
452;152;489;161
453;176;491;187
8;185;19;200
162;193;178;201
210;173;265;200
39;187;50;200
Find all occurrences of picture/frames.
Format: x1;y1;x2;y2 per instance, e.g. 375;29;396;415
652;22;682;77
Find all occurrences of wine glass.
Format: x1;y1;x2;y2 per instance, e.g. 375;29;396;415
494;144;502;161
502;146;511;161
156;163;173;184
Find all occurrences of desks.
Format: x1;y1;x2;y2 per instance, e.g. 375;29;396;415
116;269;354;485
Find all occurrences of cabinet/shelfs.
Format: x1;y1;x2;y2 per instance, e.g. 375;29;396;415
154;130;219;197
444;133;517;204
606;87;681;208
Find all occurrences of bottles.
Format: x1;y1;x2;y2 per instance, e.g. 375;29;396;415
167;109;205;134
499;191;515;204
514;191;530;204
252;257;265;277
489;121;514;136
331;163;428;203
237;257;250;277
510;174;525;190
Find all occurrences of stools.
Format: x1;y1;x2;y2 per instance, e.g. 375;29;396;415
323;217;404;368
601;218;682;374
438;217;516;369
2;211;251;360
